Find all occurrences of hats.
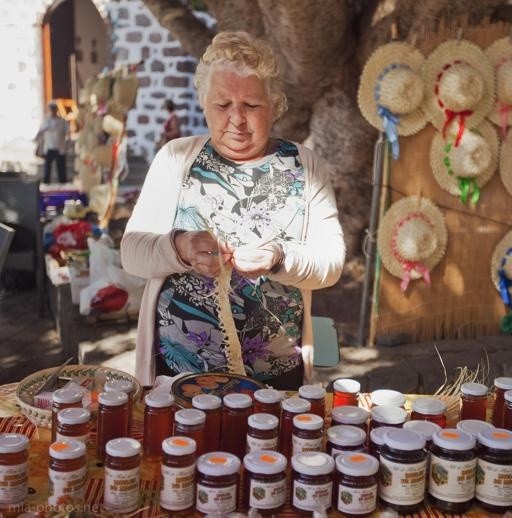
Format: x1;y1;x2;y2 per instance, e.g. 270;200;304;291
376;196;448;283
482;34;512;128
354;39;432;138
498;126;512;198
421;38;497;133
489;230;512;305
69;72;139;232
427;112;501;198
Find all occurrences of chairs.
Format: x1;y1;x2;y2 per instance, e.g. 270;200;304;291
0;222;16;274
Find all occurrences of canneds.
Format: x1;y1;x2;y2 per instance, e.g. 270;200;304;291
370;389;406;410
378;428;428;514
2;433;30;516
46;386;92;512
428;428;478;513
253;389;281;438
159;436;197;512
459;383;488;422
221;393;253;454
475;428;512;513
330;406;369;433
194;452;241;516
369;405;408;432
290;451;335;517
298;385;326;419
174;409;206;458
326;425;367;482
456;419;495;456
243;450;288;516
280;397;311;456
333;452;379;517
192;394;222;450
98;379;141;514
144;392;175;456
332;379;360;408
292;414;324;455
502;390;512;431
491;378;512;428
369;427;402;462
410;398;448;429
403;420;442;490
245;413;279;455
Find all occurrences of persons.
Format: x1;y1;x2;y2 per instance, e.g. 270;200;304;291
33;102;71;191
120;29;348;391
155;99;182;147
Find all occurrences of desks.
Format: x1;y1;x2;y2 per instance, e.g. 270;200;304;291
0;387;512;518
36;221;150;365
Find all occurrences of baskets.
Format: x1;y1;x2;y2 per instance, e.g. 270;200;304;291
15;363;144;432
